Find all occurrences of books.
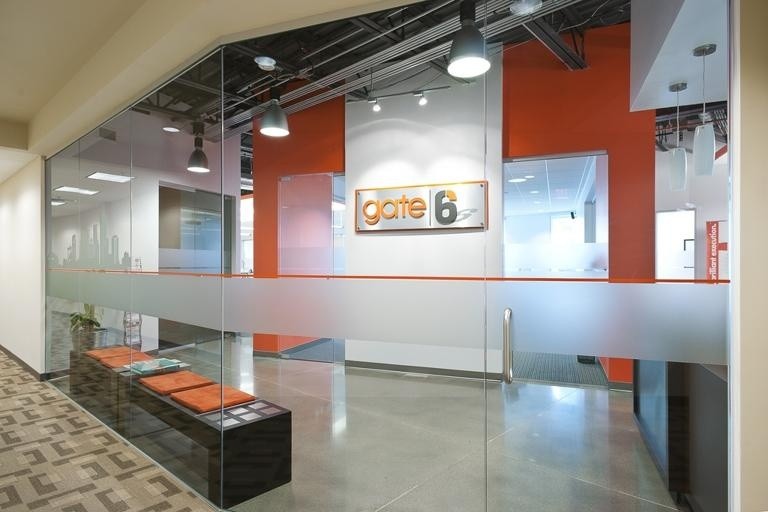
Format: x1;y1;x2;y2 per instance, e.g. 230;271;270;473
124;357;180;375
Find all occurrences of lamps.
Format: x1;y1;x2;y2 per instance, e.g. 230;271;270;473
693;44;716;177
161;112;183;133
667;83;688;193
446;0;491;79
344;85;450;112
186;137;211;174
252;55;298;73
258;87;290;137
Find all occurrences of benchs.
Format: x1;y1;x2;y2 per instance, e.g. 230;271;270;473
69;344;182;432
117;364;291;511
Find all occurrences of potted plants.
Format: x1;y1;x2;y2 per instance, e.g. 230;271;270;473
68;302;108;352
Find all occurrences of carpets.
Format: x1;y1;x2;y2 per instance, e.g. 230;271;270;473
284;339;608;389
